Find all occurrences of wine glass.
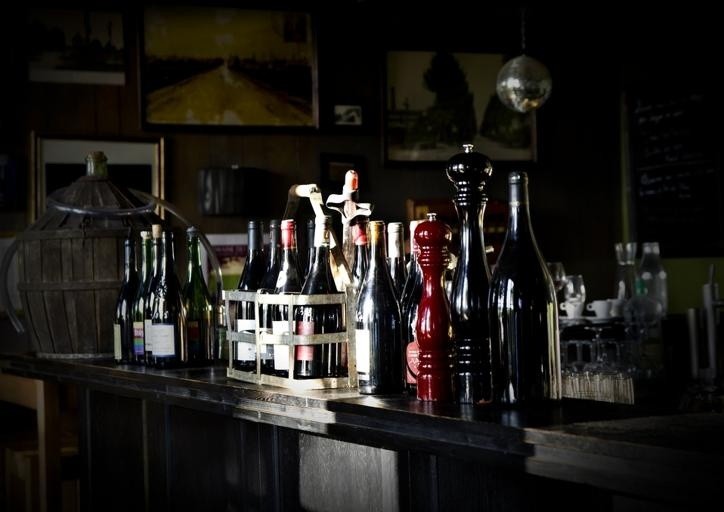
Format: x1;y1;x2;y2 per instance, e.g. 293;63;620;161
559;322;636;403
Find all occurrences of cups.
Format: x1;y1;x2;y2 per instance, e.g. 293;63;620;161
546;262;567;293
561;274;586;306
606;297;624;317
585;299;613;318
559;298;584;316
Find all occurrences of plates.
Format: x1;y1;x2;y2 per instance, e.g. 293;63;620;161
558;315;587;322
585;316;614;323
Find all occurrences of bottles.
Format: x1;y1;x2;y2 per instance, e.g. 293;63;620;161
109;223;228;369
487;167;563;405
611;240;641;298
234;213;428;397
639;240;668;316
